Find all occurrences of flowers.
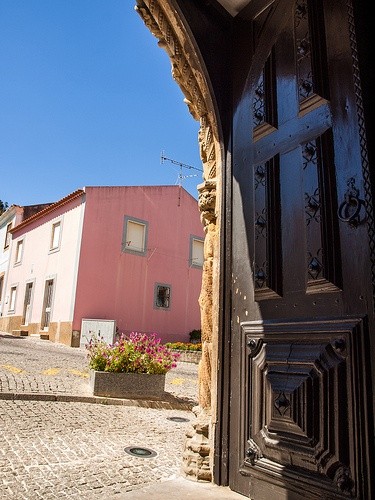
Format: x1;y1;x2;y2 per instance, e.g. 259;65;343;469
81;329;182;375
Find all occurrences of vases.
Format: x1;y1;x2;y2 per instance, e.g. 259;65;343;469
89;368;166;403
